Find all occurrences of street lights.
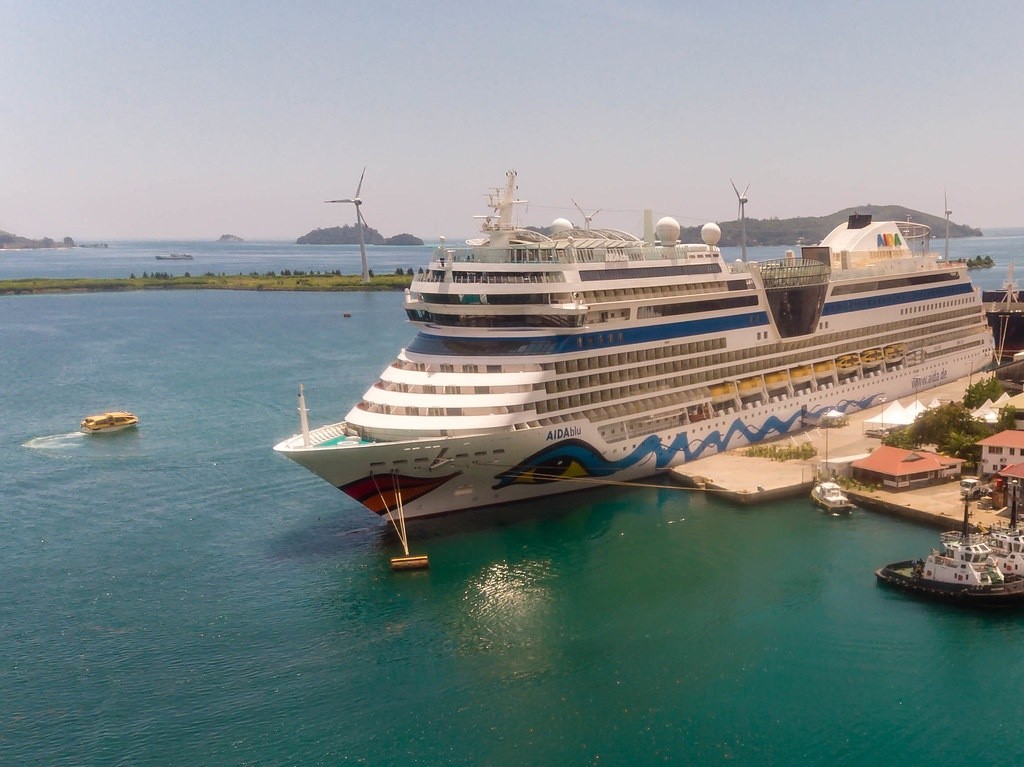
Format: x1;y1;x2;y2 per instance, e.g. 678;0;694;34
877;394;889;444
820;402;833;463
969;358;974;390
912;371;921;416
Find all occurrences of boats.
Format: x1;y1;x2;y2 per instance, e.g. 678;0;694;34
810;477;857;517
80;410;142;436
874;491;1002;607
153;252;193;260
974;482;1024;597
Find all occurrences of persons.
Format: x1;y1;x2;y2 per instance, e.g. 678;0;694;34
820;413;827;428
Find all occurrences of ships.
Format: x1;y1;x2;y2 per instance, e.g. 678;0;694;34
265;164;1002;533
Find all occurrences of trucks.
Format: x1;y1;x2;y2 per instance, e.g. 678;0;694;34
960;478;992;500
863;428;886;436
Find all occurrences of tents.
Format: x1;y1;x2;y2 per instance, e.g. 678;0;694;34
863;398;941;435
